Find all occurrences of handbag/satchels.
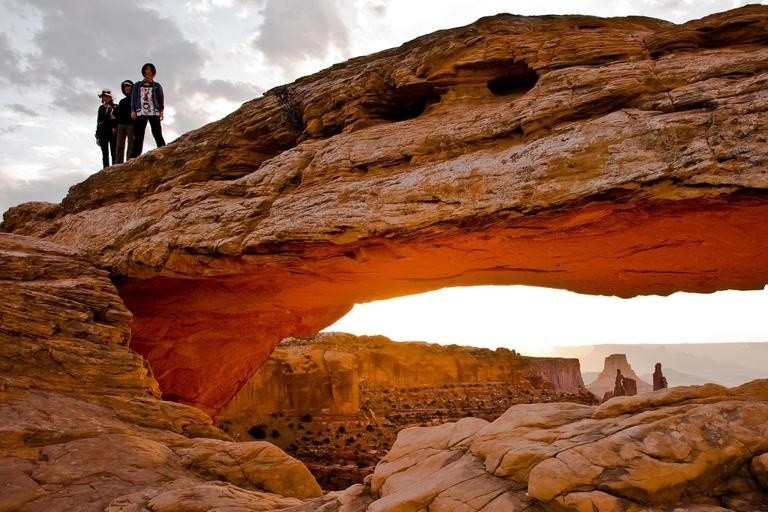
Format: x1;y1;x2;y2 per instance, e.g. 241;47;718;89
96;132;102;146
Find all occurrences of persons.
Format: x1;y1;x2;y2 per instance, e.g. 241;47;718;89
96;89;119;169
112;79;135;165
130;63;166;162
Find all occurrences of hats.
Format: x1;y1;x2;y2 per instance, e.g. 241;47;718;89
98;88;116;101
123;81;133;87
